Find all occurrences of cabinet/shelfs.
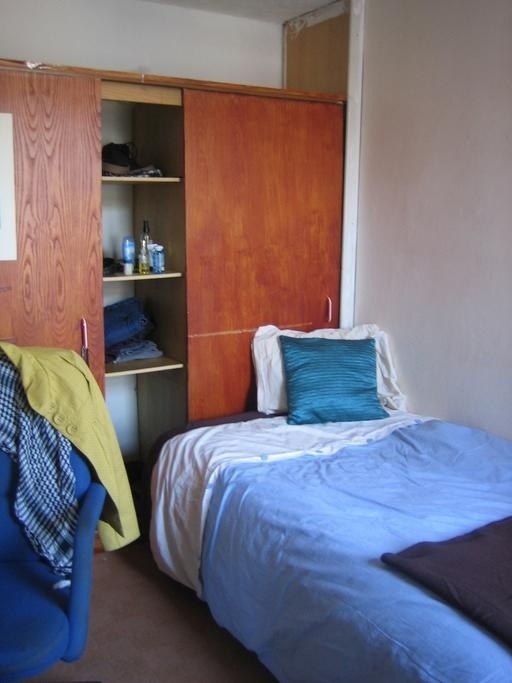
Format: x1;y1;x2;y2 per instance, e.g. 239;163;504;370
0;57;349;548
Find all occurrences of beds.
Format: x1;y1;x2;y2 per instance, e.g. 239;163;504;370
150;401;512;683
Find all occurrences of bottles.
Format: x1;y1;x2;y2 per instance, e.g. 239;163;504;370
141;219;150;249
151;245;167;273
122;236;135;276
139;240;149;274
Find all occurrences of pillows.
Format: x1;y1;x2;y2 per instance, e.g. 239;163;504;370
250;322;408;415
276;333;392;425
